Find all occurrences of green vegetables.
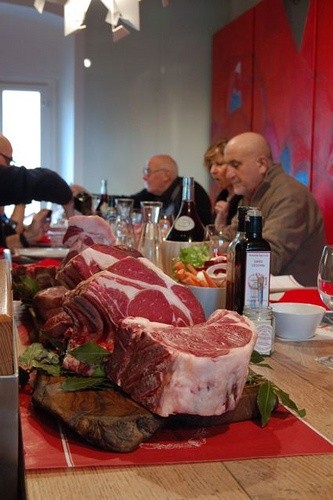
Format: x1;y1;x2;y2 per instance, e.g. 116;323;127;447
172;243;210;274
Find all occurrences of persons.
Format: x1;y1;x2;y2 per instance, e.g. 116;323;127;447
223;133;326;286
0;136;244;252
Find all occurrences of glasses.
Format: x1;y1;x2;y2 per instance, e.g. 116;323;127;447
0;152;13;165
142;168;169;175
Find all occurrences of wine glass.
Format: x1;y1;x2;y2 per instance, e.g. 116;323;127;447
318;245;333;364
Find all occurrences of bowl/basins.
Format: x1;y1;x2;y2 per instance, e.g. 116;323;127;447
270;302;327;339
175;278;227;321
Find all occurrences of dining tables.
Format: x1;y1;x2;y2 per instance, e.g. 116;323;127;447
8;322;333;500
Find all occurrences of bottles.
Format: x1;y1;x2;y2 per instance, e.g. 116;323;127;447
114;176;276;359
74;194;90;209
95;179;112;219
